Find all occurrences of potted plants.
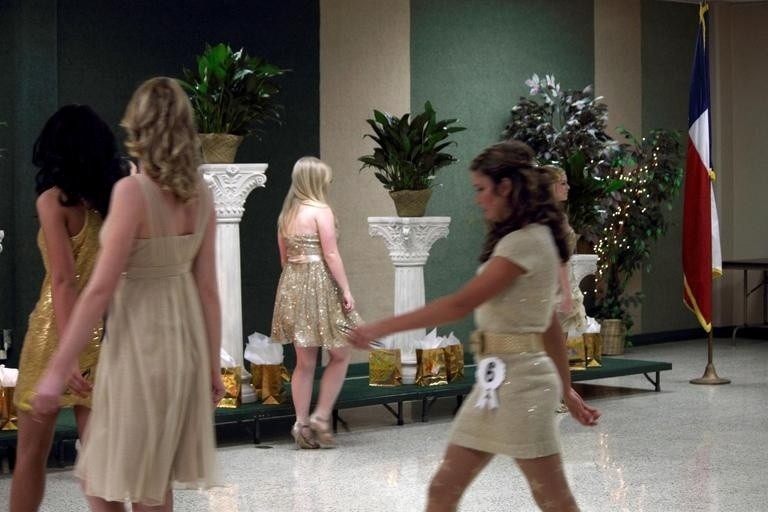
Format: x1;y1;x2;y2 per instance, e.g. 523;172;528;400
497;71;686;357
353;99;470;218
170;38;296;162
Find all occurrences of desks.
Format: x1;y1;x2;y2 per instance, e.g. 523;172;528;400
0;353;672;476
722;258;768;345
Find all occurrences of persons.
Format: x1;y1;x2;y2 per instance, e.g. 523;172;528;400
269;154;367;450
348;139;600;512
11;105;130;510
539;164;576;414
27;78;227;512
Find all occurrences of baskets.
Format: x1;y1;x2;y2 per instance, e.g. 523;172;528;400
599;318;626;356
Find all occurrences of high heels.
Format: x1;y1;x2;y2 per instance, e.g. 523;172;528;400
290;418;339;450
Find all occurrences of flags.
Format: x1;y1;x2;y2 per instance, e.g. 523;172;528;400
680;8;724;334
